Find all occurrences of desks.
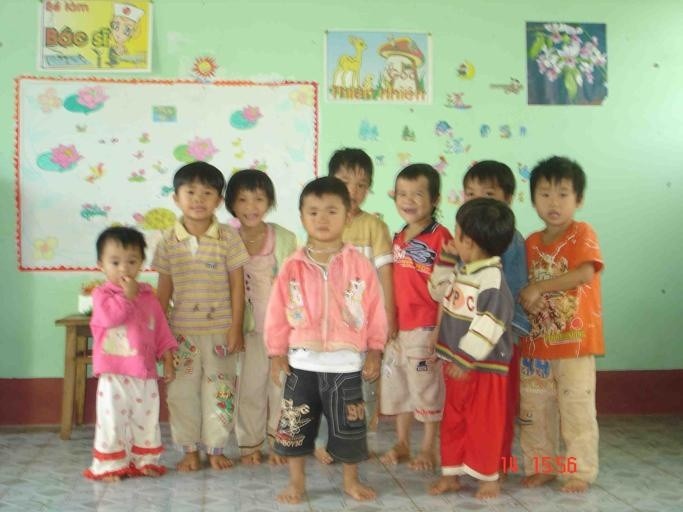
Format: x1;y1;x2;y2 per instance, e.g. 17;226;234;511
54;311;99;439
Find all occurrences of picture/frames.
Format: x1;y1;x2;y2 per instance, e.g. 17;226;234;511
322;28;433;107
35;1;155;73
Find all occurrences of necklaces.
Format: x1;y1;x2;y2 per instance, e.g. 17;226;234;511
238;229;267;244
306;245;340;260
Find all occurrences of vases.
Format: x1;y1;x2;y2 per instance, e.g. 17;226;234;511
78;296;94;316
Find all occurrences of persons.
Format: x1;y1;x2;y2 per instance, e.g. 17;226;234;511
152;161;249;470
224;169;296;465
519;156;605;491
380;163;456;471
463;162;526;479
313;149;393;462
87;227;176;483
427;196;515;500
263;176;390;503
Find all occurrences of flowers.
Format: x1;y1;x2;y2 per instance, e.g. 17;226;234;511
75;277;107;296
526;23;606;101
